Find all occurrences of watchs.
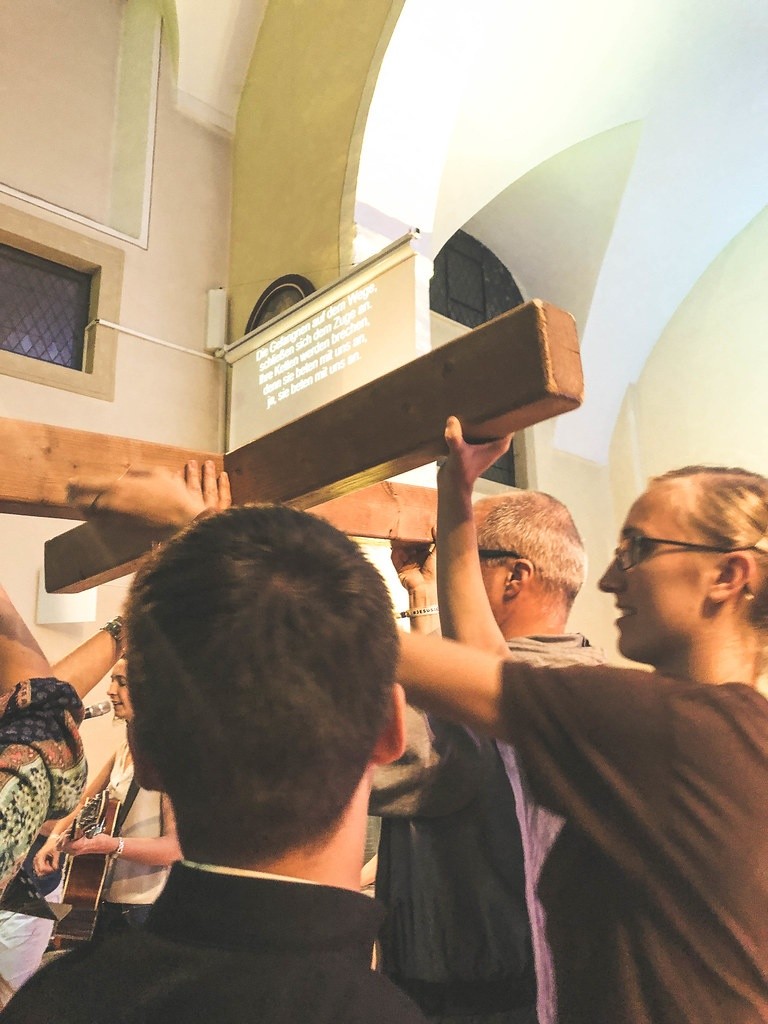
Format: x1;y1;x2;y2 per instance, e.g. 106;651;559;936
99;618;135;656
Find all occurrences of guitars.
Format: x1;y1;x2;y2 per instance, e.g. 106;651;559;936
51;789;121;944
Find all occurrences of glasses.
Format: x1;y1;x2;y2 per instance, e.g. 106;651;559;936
613;536;757;570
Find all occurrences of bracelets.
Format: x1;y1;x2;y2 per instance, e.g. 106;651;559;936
107;835;124;863
394;605;444;620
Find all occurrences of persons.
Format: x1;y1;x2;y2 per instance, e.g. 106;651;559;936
0;410;768;1024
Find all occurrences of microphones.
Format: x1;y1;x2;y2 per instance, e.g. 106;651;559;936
84;701;112;719
